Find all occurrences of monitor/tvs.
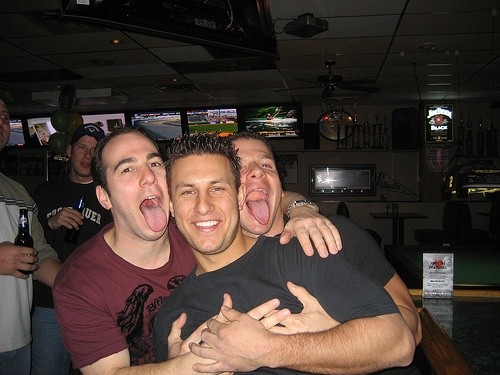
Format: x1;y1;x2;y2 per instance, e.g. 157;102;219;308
5;116;28;146
242;102;303;139
79;111;129;136
127;109;184;142
24;114;58;145
185;106;242;139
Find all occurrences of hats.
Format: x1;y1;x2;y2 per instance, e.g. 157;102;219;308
70;123;105;144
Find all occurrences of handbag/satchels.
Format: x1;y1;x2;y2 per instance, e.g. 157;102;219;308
414;306;472;375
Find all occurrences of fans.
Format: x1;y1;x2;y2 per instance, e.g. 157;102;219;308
273;60;384;93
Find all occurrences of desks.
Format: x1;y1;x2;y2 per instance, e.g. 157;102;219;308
371;213;426;246
384;244;499;290
408;288;500;374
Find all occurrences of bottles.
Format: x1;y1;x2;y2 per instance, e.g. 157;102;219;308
14;208;34;274
64;195;86;244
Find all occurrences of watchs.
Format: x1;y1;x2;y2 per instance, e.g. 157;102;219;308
286;199;320;218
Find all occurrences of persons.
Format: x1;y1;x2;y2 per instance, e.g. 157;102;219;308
0;94;60;375
51;125;341;375
150;135;419;375
232;131;422;347
30;123;114;375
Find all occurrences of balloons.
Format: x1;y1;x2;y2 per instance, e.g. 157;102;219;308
49;133;65;153
64;132;75;146
67;111;82;132
51;111;68;131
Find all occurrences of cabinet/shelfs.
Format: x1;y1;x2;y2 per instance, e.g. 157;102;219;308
278;147;427;204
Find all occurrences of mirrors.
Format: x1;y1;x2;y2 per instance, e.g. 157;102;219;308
310;164;379;197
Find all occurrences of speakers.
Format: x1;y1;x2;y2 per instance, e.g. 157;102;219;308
391;106;419;152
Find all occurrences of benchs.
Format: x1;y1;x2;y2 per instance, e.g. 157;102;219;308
414;200;499;243
339;202;381;248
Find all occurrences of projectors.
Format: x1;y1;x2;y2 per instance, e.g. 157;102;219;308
284;14;330;39
